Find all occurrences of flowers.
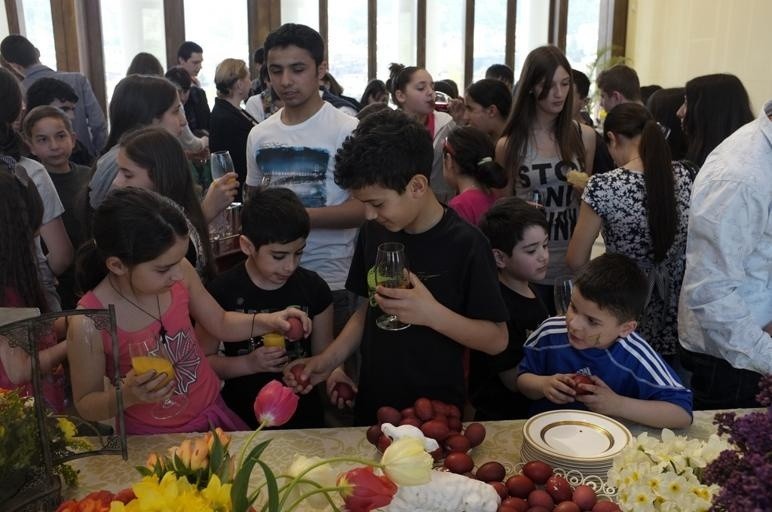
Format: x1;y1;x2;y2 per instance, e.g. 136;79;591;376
56;379;434;512
608;374;772;512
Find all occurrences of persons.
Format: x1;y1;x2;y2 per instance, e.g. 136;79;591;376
1;35;756;438
244;23;366;342
675;99;771;409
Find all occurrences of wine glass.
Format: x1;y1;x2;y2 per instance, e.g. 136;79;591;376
375;242;411;331
431;91;455;105
210;151;241;210
554;275;577;315
527;190;545;209
657;122;671;141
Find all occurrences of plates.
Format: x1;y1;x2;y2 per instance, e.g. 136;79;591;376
519;410;632;497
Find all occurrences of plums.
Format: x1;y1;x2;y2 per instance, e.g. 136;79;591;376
367;398;622;512
570;375;595;396
290;365;310;389
285;318;304;341
332;382;355;401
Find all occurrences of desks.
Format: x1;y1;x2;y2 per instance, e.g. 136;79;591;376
52;408;772;512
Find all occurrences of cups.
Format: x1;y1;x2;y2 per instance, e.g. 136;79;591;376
261;328;288;368
129;335;175;393
